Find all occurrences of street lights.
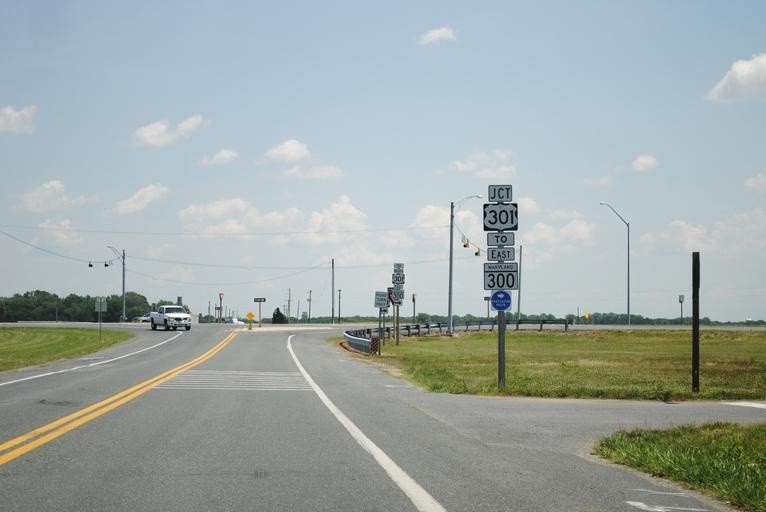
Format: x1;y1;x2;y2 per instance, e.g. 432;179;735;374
678;294;684;327
106;243;126;321
219;293;224;321
599;201;630;326
338;289;342;319
447;194;483;335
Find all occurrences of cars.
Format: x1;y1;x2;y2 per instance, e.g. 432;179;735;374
132;313;150;322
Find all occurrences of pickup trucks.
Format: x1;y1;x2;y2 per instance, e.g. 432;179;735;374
149;305;192;331
